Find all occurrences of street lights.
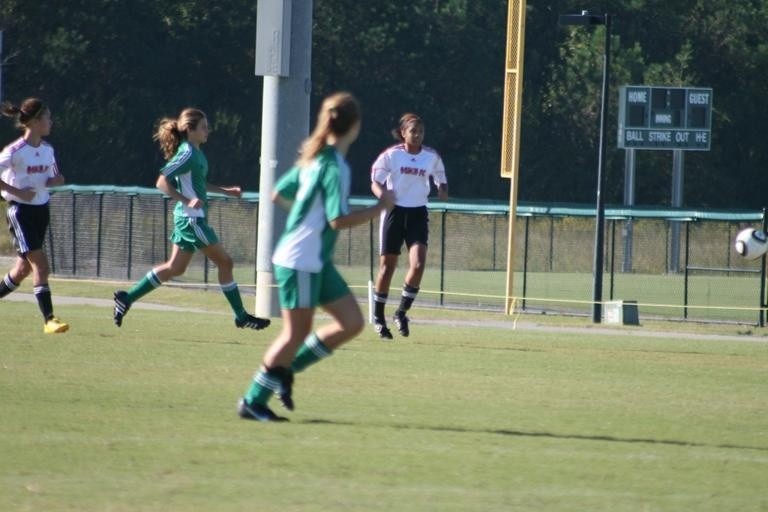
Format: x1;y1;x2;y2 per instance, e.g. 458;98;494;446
558;10;613;322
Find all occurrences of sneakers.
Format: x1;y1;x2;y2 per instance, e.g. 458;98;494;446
373;316;393;340
277;374;295;412
43;318;70;335
237;398;290;424
234;313;272;330
113;290;129;327
394;312;410;338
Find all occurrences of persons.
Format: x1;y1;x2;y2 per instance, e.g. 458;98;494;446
369;112;448;338
0;96;69;333
237;89;396;421
113;105;270;330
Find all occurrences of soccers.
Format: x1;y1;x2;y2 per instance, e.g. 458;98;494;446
735;228;768;261
